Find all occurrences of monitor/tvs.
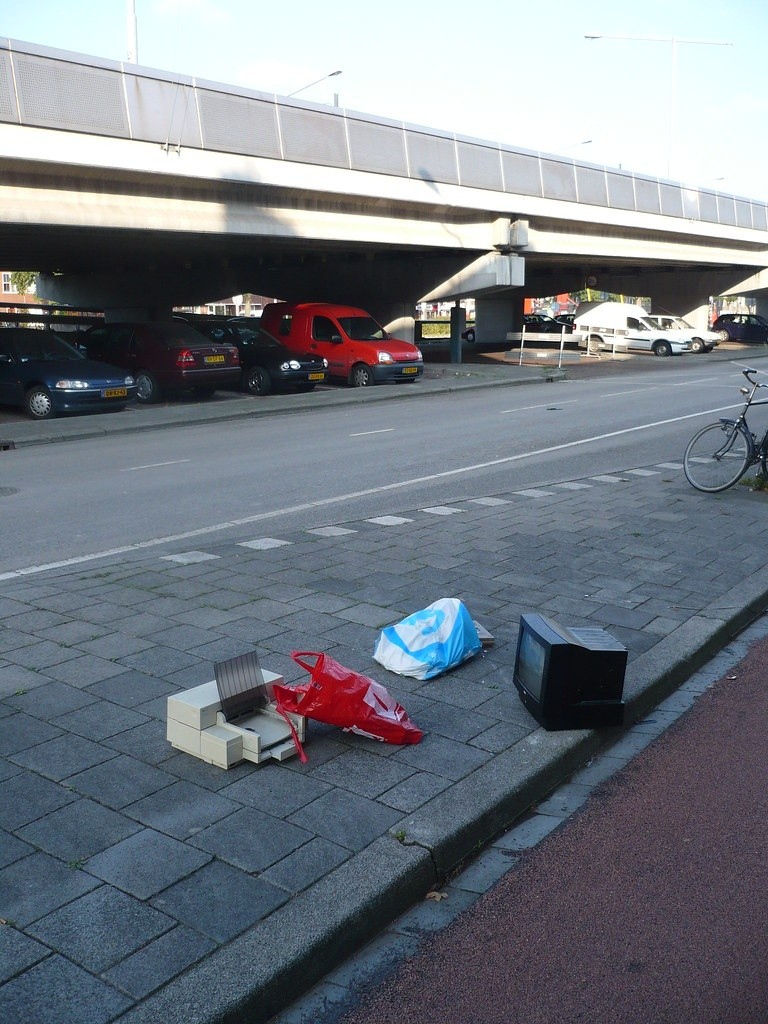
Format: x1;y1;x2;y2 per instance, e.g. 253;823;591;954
513;613;628;731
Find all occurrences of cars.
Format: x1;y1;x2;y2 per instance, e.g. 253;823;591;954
0;325;139;421
711;314;768;346
198;319;330;396
68;321;242;406
639;313;721;354
461;313;576;344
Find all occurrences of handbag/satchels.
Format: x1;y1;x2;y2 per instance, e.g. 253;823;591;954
272;651;423;763
371;598;483;681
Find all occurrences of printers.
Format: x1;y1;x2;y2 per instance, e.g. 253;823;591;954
167;650;307;770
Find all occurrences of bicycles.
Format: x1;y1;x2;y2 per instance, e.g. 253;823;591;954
683;368;767;493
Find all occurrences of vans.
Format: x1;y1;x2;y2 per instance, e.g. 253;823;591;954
261;302;424;389
573;301;694;358
